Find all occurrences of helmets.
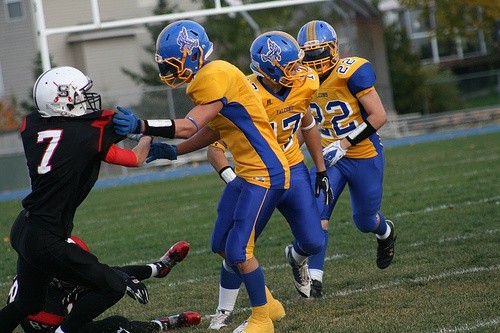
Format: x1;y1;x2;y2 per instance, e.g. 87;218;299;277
32;65;88;115
155;20;214;76
249;31;305;84
297;21;338;62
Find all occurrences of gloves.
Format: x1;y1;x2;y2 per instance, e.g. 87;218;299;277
112;105;141;136
146;143;178;164
126;278;149;305
315;170;334;206
321;140;349;168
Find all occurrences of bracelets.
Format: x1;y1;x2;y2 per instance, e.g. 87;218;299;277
184;116;200;131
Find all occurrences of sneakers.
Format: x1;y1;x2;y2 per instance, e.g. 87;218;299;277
285;244;312;299
157;311;201;332
151;241;189;277
310;280;324;299
208;307;234;331
376;221;394;268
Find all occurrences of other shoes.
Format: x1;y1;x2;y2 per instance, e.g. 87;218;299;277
232;305;272;333
268;300;285;321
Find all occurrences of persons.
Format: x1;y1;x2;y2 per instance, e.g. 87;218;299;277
111;20;290;333
296;20;396;300
7;235;201;333
207;31;335;331
0;65;153;333
